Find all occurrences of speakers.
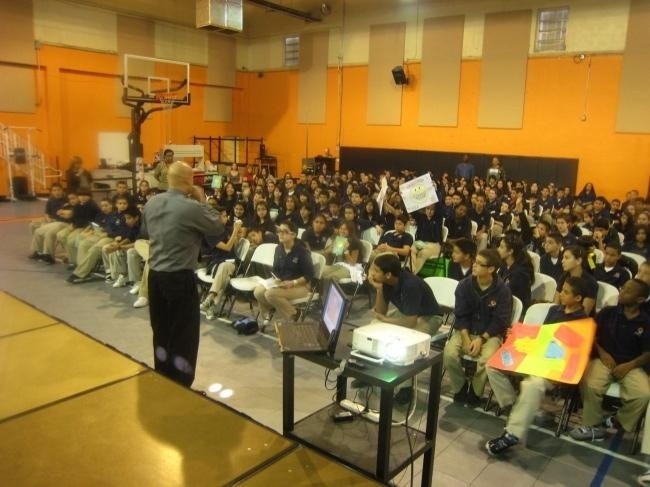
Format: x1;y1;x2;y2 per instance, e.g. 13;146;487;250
392;65;406;84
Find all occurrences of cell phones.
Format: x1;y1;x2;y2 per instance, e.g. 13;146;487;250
233;216;239;222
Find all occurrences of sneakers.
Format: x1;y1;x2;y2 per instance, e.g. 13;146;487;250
485;430;520;457
133;297;149;308
453;377;469;403
350;378;369;389
289;307;304;323
104;274;129;288
199;297;218;321
262;307;277;327
466;381;481;408
60;257;92;284
395;387;413;405
26;249;56;265
569;415;615;443
129;281;141;295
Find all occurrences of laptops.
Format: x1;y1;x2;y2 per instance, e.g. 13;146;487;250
273;278;349;353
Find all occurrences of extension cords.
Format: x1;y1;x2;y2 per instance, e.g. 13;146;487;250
339;398;380;424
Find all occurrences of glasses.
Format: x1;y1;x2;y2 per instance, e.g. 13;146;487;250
471;258;494;269
276;228;296;235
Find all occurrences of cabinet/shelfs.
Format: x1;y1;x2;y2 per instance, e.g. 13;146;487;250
280;318;447;486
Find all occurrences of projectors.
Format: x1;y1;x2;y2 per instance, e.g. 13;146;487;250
350;319;431;366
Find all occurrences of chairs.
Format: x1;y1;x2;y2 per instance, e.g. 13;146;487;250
471;205;543;246
618;232;625;245
580;227;592;236
385;218;449;278
196;209;383;320
423;250;650;455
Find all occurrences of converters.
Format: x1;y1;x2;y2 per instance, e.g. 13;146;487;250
334;410;353;421
